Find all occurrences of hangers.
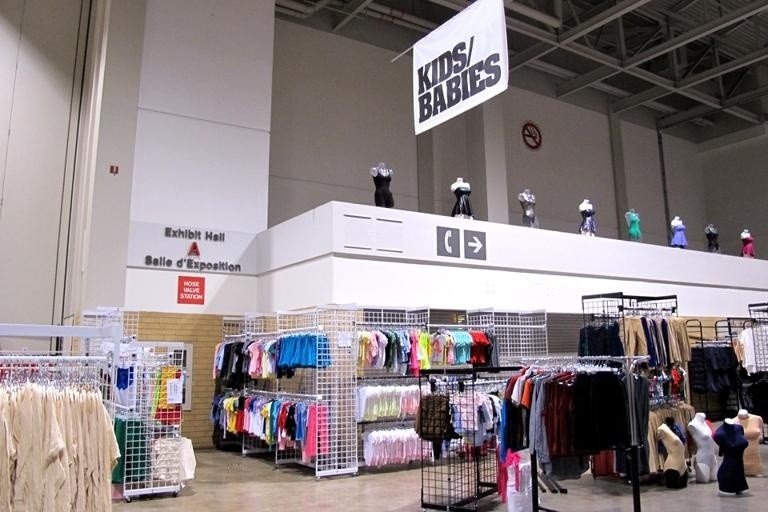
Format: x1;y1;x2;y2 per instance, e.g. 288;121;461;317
509;355;613;377
